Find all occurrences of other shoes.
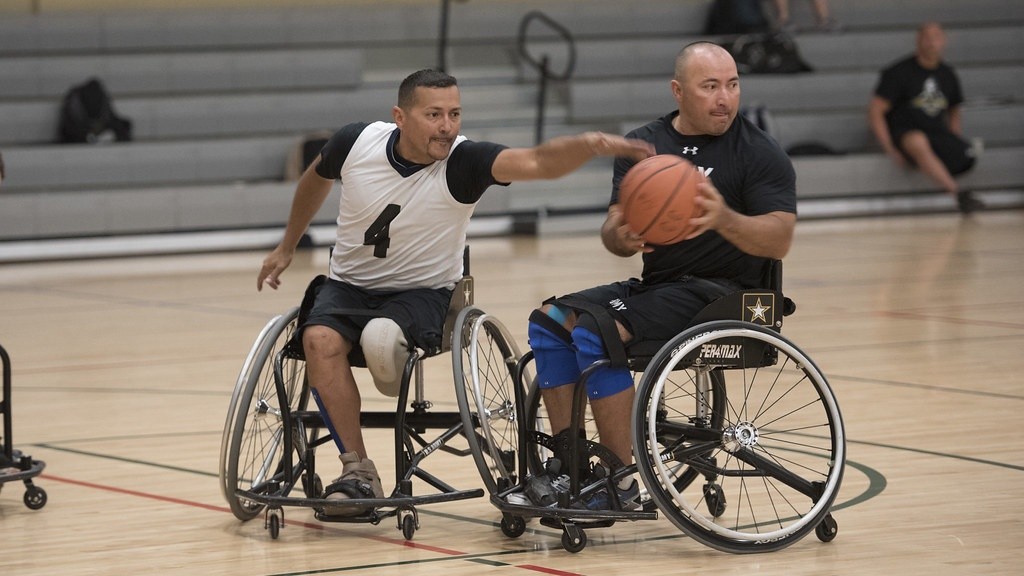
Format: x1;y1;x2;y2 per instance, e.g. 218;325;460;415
956;182;986;214
322;450;385;516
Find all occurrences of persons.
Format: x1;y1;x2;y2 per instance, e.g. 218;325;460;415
506;44;797;510
777;0;840;34
258;68;654;522
869;25;990;210
705;0;781;71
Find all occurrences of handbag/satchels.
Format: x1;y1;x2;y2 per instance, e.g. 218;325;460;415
55;78;132;146
726;29;813;72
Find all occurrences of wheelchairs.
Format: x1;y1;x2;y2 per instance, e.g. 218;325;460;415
490;284;847;557
217;245;536;535
0;347;47;509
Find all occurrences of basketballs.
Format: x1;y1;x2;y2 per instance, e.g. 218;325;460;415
619;153;708;246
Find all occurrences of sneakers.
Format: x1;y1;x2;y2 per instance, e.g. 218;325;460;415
571;478;642;510
502;460;611;508
